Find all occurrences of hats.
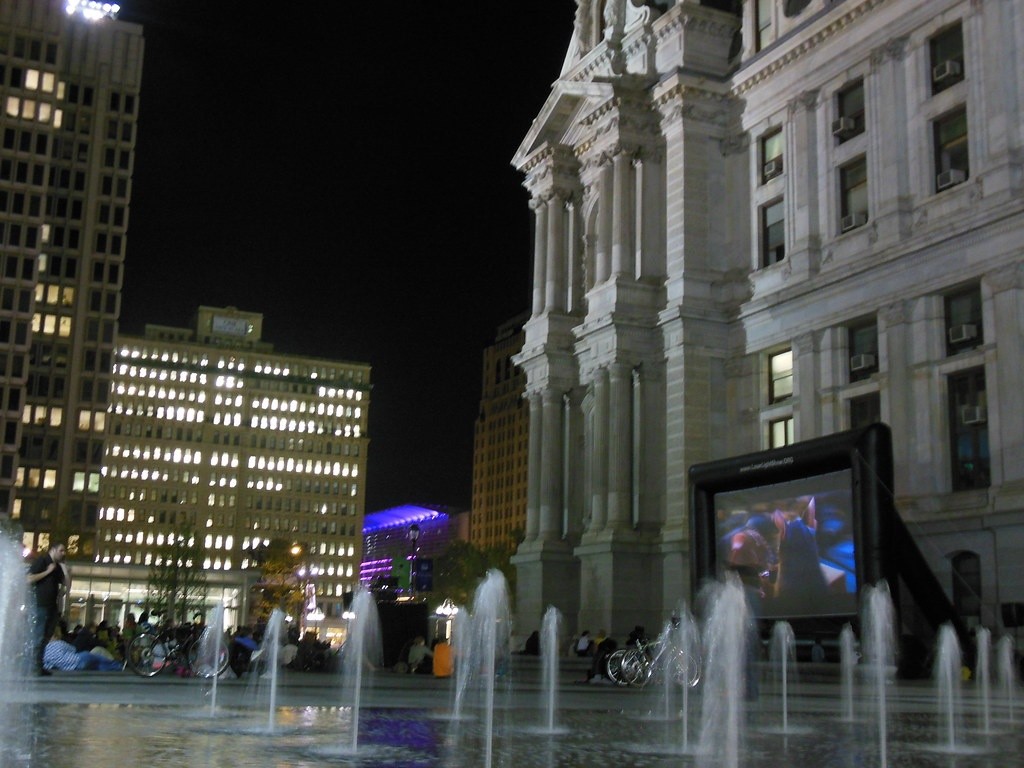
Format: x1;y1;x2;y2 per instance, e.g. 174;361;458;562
435;634;448;643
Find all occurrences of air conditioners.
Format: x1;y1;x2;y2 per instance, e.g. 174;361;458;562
963;406;988;424
832;117;854;135
851;354;875;371
841;213;865;232
933;60;959;82
765;161;780;176
949;324;976;343
938;169;965;190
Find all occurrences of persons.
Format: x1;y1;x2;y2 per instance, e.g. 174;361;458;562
43;612;381;672
395;634;508;682
18;542;70;677
524;624;651;682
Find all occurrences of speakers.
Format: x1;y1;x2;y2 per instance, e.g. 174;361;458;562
411;557;434;592
1001;603;1024;628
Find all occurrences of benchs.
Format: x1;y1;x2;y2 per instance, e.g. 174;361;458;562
509;654;593;683
761;662;897;685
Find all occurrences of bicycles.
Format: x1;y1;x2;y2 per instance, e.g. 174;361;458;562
128;607;232;678
605;616;702;689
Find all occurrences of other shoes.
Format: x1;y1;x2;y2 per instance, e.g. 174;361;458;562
34;668;52;677
120;658;127;671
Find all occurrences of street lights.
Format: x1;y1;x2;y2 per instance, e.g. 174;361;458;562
407;522;421;605
